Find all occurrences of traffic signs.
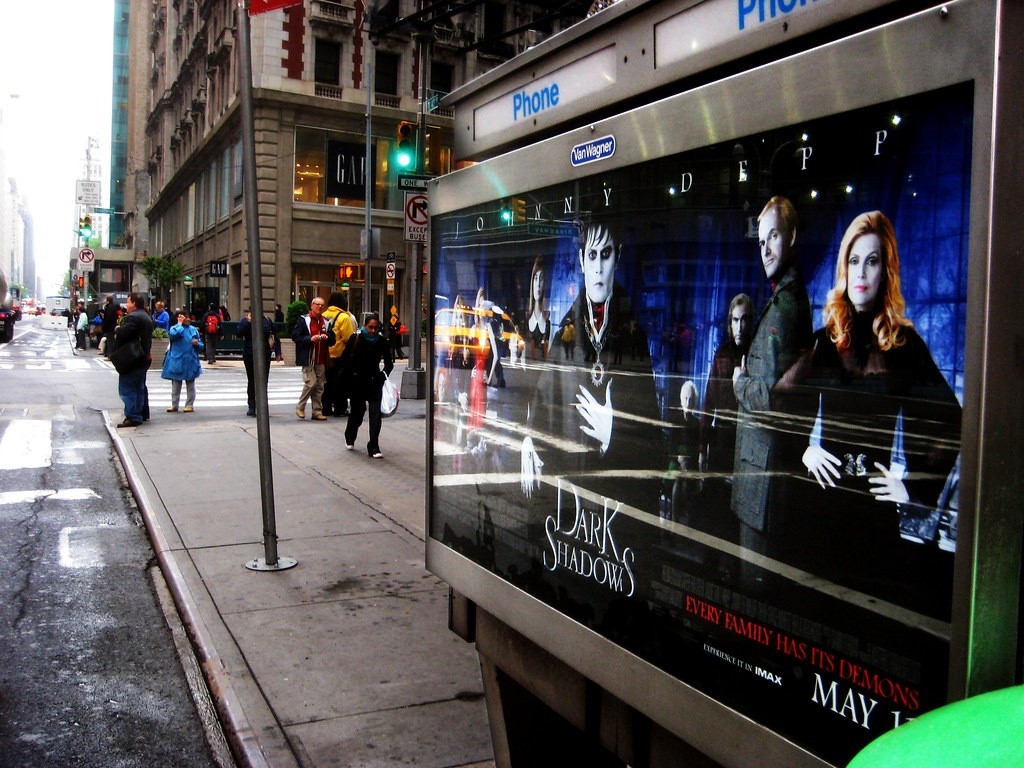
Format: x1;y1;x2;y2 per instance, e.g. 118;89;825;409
93;208;115;215
76;179;101;205
397;176;443;192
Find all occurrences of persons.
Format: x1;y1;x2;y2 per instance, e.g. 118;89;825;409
236;306;280;416
660;381;700;538
322;292;358;416
390;314;405;360
731;196;813;586
111;292;154;428
274;304;284;322
341;311;393;459
292;297;336;421
75;295;230;365
161;310;204;412
436;199;661;605
698;293;754;472
767;211;962;588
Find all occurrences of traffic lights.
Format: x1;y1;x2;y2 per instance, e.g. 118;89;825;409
396;122;415;170
76;217;85;239
338;267;345;279
81;215;93;240
343;264;360;281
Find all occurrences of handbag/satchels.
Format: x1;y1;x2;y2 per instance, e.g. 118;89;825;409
268;334;275;349
380;369;400;417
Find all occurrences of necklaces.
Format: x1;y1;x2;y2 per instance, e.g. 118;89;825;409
585;292;612;386
313;313;323;324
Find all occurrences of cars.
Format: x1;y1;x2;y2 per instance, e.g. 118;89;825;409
434;302;525;370
50;307;70;327
0;299;45;344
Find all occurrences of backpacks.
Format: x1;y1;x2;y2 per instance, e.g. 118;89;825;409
206;313;218;333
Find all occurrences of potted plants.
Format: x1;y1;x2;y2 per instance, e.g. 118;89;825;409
150;326;170;371
279;300;310;365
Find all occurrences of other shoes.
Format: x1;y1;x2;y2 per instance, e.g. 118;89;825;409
336;410;350;417
183;407;192;412
311;414;327;420
118;418;141;428
323;411;334;416
297;409;305;417
370;450;383;458
247;408;255;415
167;408;177;412
143;418;147;421
345;439;354;449
